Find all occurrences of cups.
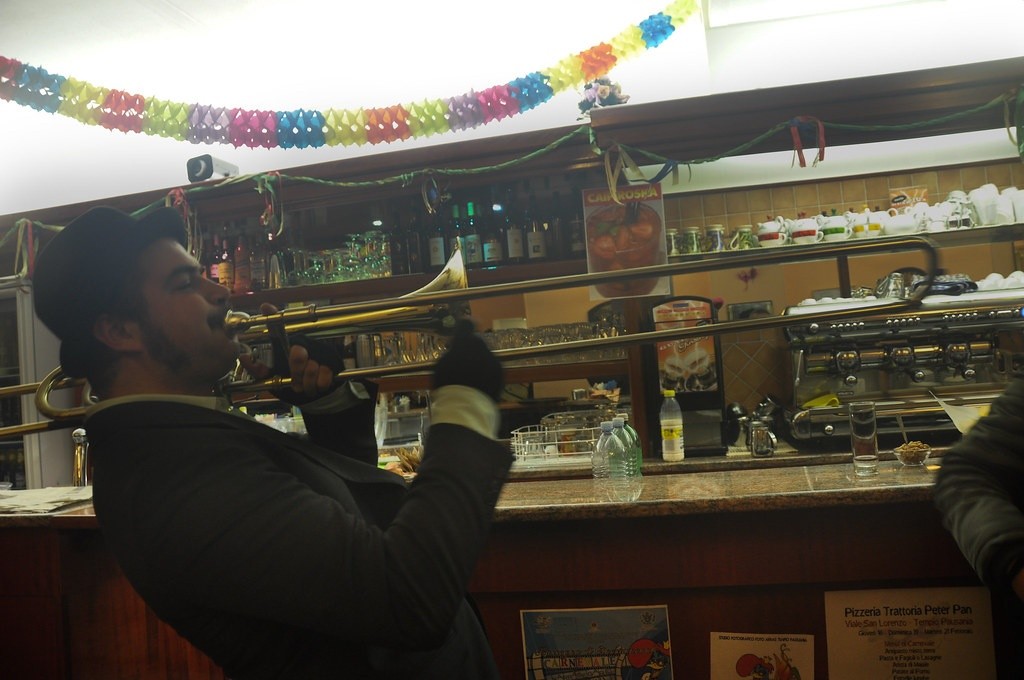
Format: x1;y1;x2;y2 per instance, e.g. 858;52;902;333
849;401;880;477
661;359;684;390
884;270;906;299
481;321;597;350
665;225;755;255
728;394;781;452
685;352;715;388
946;184;1024;230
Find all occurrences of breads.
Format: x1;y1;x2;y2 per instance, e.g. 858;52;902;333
664;350;708;378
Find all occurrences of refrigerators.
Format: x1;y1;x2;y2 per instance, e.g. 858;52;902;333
0;275;76;489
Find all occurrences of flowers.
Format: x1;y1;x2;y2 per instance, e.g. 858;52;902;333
577;77;630;120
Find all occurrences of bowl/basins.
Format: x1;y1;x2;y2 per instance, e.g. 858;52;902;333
893;446;931;466
0;482;12;496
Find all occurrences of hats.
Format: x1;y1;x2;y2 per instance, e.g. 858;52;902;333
32;203;188;378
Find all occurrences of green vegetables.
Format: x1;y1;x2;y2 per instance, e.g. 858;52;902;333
596;219;624;237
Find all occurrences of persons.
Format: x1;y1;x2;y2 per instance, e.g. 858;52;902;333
32;205;517;680
930;370;1024;600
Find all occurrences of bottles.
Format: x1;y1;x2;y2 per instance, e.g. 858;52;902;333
203;219;287;297
0;395;26;488
590;413;644;502
659;390;684;461
389;188;586;275
382;330;444;367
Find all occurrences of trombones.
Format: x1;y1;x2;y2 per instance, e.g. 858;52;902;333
0;232;945;444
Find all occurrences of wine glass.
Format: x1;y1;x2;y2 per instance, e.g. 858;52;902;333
286;229;393;286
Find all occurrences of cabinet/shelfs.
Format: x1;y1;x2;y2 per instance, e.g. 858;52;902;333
0;283;86;491
0;58;1024;461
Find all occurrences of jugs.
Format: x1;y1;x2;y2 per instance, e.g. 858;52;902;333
757;202;950;247
752;426;778;458
